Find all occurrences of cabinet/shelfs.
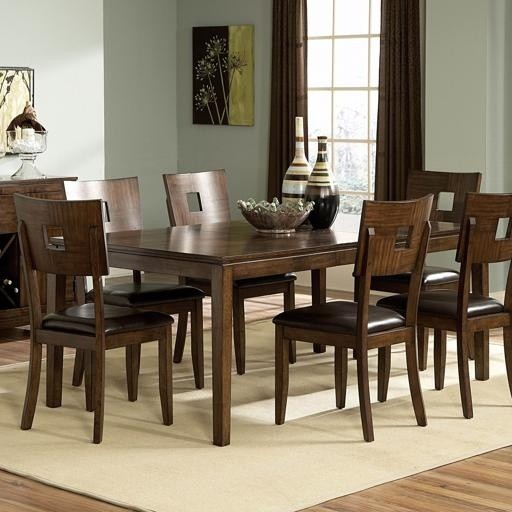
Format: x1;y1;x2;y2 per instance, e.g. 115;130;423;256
0;179;74;328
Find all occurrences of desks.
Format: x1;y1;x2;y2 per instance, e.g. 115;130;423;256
107;212;490;448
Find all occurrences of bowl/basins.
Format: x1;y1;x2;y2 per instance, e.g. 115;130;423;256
238;199;312;234
245;237;308;258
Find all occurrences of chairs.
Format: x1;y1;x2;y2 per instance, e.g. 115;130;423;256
162;168;298;375
271;193;436;442
63;175;206;401
377;190;512;418
13;191;172;445
353;169;482;359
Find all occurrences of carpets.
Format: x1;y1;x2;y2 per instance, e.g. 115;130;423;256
0;317;512;512
